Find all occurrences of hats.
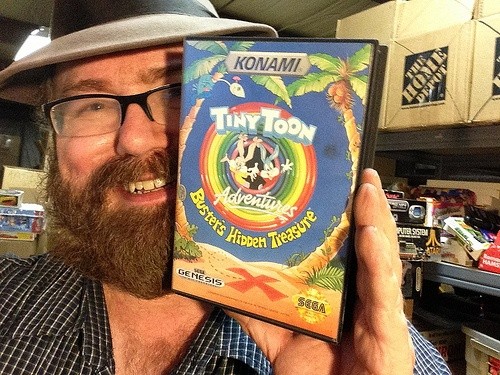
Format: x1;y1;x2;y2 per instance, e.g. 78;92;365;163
2;1;279;106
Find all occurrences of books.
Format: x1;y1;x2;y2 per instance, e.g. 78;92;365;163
172;36;388;347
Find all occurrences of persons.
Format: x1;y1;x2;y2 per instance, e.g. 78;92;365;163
0;0;452;375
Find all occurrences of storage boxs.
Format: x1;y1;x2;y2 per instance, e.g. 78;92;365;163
460;316;500;375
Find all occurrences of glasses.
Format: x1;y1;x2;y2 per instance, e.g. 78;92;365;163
41;83;180;138
